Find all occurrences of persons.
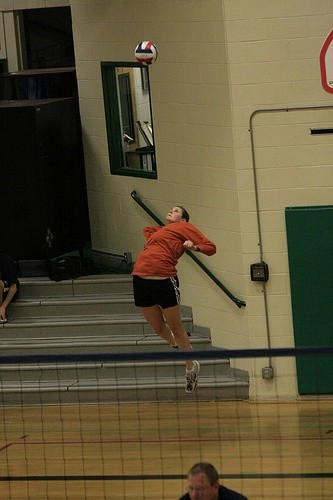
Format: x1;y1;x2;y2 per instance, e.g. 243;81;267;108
0;254;21;324
178;463;249;499
132;205;216;394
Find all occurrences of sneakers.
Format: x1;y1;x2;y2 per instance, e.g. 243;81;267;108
170;330;178;352
0;316;8;324
185;360;200;394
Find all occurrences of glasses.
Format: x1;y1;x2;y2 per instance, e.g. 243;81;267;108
184;485;212;493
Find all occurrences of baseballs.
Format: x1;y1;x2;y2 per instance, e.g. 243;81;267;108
135;41;158;66
